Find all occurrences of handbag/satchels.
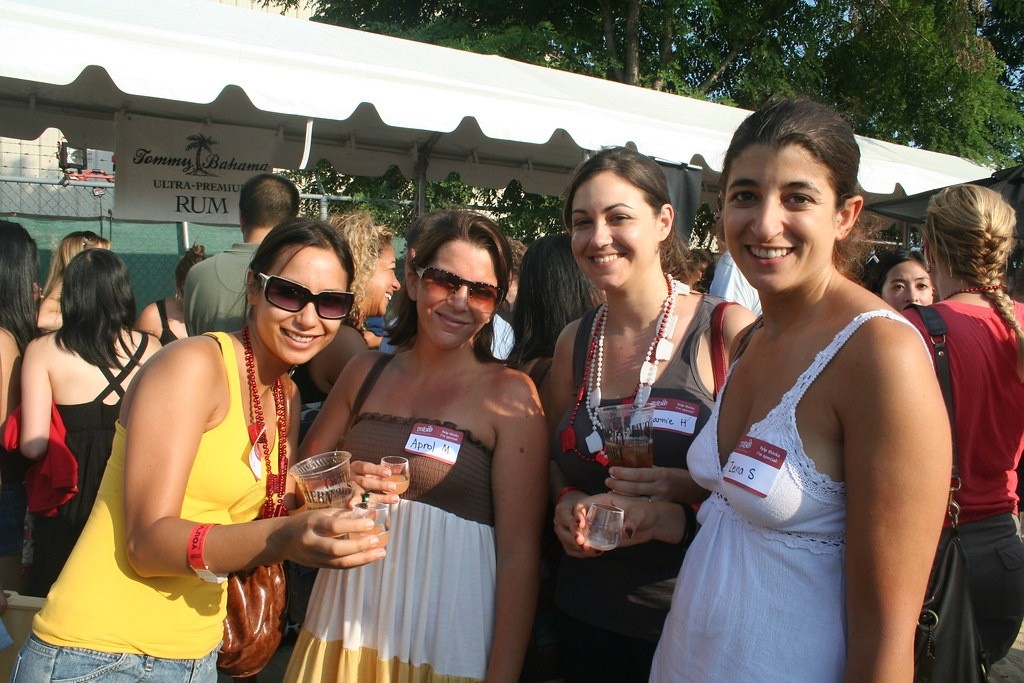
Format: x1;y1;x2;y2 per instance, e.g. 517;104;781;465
913;537;992;683
218;559;291;678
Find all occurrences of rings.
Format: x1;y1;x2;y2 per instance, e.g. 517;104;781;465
649;497;651;503
362;491;371;503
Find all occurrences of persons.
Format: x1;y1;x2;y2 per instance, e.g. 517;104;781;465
893;182;1024;683
292;205;403;588
0;218;210;598
376;209;933;387
519;143;762;682
289;207;550;683
184;174;299;336
9;214;387;683
651;96;952;683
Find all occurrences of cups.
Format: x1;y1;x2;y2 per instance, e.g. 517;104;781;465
349;502;391;549
583;503;623;550
290;451;352;538
598;403;653;469
380;456;410;494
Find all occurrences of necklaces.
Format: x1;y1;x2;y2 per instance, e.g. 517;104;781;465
944;286;1003;301
241;319;289;519
561;270;690;462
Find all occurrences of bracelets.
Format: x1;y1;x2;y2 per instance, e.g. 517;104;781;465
187;523;229;585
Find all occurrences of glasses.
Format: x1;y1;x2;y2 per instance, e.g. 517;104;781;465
717;195;723;213
82;231;98;247
251;270;354;320
412;262;504;311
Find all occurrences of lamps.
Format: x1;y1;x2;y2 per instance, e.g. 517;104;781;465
59;142;87;176
